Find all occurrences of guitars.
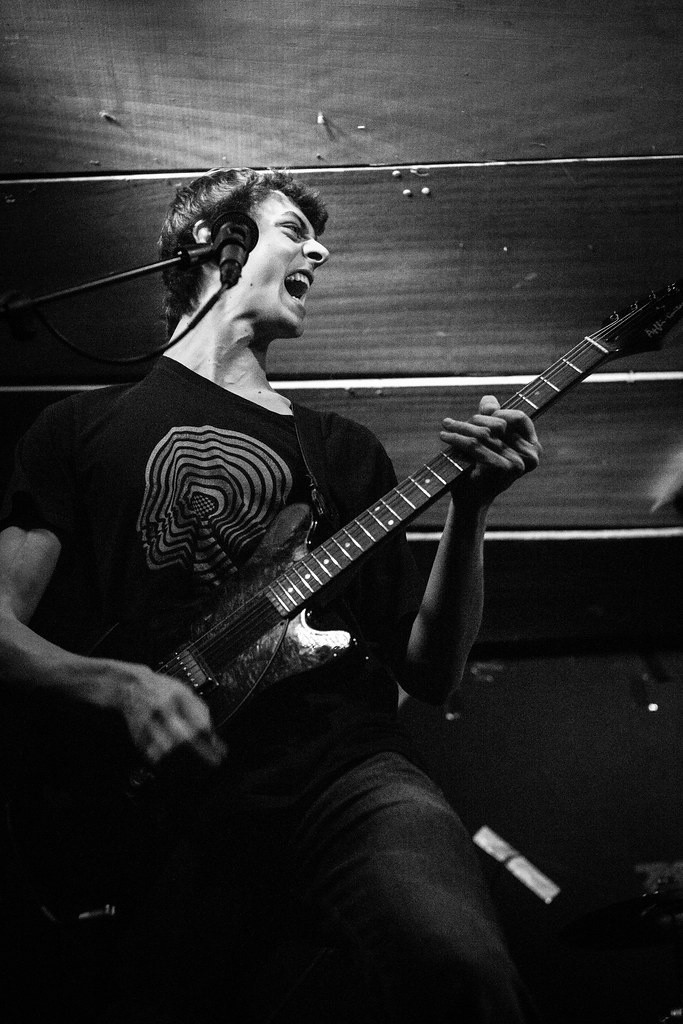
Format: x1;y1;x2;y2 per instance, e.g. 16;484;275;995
68;278;681;790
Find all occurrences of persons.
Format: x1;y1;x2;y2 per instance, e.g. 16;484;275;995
0;165;544;1023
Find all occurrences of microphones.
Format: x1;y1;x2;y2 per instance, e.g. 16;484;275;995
211;210;259;287
